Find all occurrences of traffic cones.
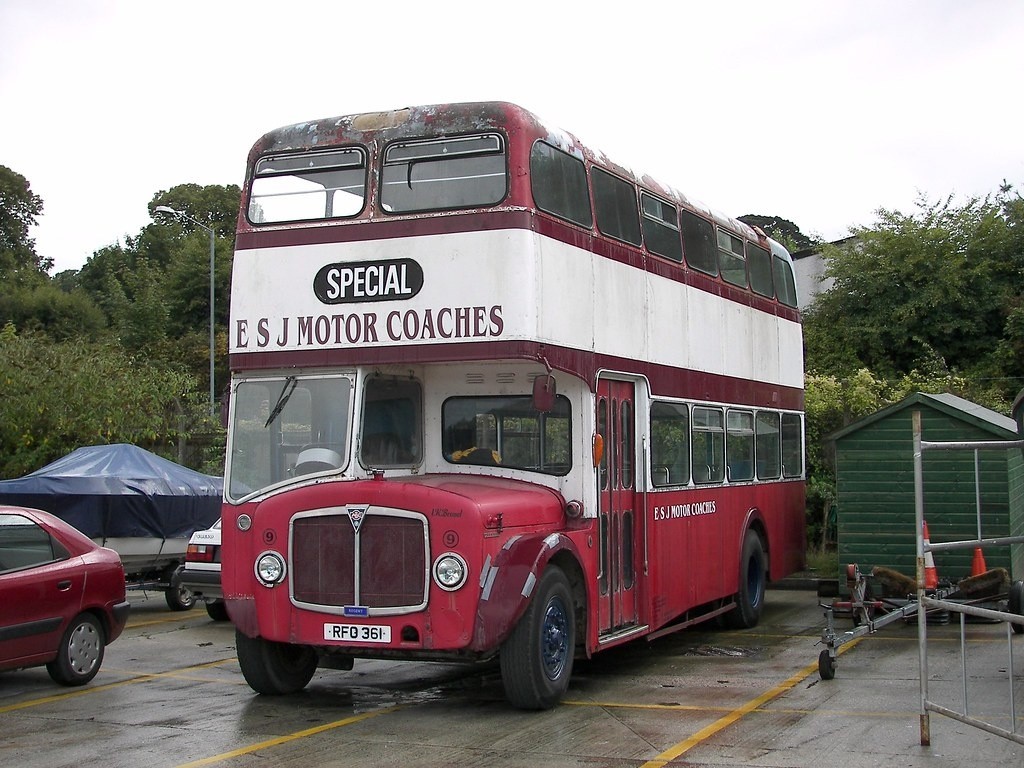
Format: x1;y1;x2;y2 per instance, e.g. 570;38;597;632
922;519;940;589
973;547;987;577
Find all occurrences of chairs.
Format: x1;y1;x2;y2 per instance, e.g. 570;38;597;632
603;464;730;487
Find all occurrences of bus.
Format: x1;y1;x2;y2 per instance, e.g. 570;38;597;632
213;101;810;710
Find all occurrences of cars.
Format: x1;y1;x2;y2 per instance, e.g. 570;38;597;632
0;506;131;686
179;517;229;621
